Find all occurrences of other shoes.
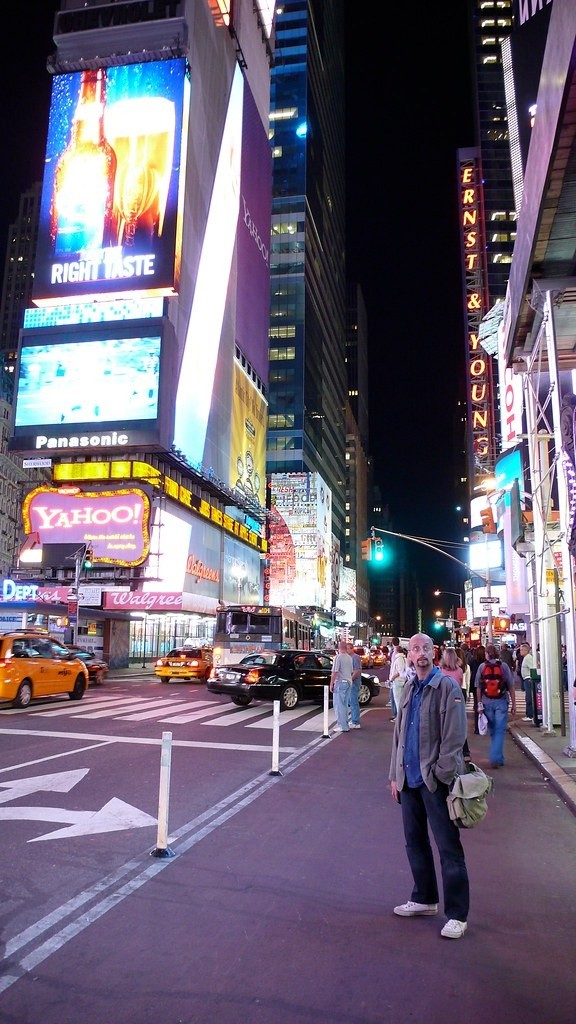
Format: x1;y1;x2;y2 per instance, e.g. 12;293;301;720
464;756;471;762
333;725;350;732
490;760;500;770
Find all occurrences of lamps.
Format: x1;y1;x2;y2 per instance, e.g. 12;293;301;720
170;444;279;529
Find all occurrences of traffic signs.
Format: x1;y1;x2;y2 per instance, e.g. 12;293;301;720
480;596;500;603
66;593;83;601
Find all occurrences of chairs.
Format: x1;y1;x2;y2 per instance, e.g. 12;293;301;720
303;658;317;669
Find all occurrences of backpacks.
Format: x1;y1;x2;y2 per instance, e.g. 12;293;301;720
480;660;506;698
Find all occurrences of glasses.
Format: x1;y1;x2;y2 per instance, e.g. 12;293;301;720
408;645;434;652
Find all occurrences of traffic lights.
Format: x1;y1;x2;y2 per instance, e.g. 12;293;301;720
451;638;454;644
374;538;383;561
493;617;510;629
435;621;440;631
480;507;497;534
361;539;371;560
84;547;94;569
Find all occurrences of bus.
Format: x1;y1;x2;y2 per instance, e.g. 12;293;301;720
209;604;315;671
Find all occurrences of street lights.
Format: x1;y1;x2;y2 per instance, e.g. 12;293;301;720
436;609;453;639
366;616;381;648
434;589;463;637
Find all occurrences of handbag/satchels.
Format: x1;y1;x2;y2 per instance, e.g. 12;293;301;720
446;762;496;829
477;702;488;736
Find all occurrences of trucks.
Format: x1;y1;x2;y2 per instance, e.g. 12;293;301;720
381;636;410;651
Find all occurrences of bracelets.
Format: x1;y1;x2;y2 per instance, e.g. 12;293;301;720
390;679;393;683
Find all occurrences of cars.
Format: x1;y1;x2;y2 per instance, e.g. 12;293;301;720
154;643;213;685
309;644;394;670
208;647;382;711
0;628;109;708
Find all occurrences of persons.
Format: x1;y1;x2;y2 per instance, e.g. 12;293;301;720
501;642;568;725
388;633;469;939
475;645;516;768
388;637;406;722
346;642;361;728
330;642;353;731
573;677;576;701
432;644;486;734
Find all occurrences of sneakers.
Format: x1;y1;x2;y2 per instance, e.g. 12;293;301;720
440;919;468;938
394;900;438;916
521;716;533;722
348;720;361;728
